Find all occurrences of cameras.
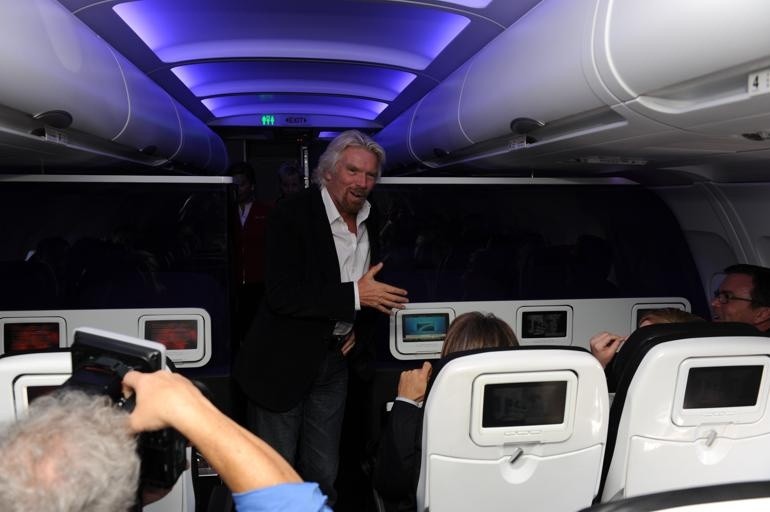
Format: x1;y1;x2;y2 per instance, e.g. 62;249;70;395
56;327;213;493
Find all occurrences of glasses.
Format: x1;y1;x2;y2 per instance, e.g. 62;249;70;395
713;290;752;303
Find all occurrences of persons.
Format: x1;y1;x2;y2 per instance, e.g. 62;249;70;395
590;307;710;393
270;160;304;208
705;263;770;336
0;365;333;512
224;164;273;356
229;128;410;507
385;311;521;512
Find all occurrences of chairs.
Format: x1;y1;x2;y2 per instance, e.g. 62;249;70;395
0;350;211;512
415;344;610;511
599;321;769;506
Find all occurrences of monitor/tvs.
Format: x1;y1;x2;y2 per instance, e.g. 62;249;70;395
1;316;68;354
137;314;204;364
516;306;573;350
631;301;687;333
394;308;457;355
670;356;770;427
470;370;577;445
14;374;74;419
